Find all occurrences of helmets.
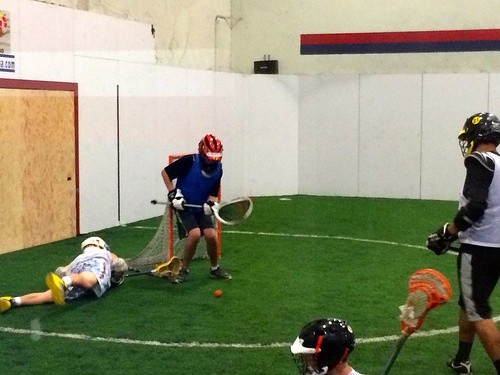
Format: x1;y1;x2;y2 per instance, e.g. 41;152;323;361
81;237;111;251
198;134;224;167
458;112;500;158
290;318;355;371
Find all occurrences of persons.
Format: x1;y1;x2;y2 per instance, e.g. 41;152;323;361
162;134;232;283
290;318;363;375
0;237;128;312
427;113;500;375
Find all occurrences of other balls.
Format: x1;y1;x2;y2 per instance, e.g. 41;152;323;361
214;290;222;297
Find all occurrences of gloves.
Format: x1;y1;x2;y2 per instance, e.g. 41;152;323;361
203;200;220;215
166;186;187;211
426;222;459;255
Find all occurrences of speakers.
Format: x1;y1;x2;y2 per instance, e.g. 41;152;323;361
254;60;278;74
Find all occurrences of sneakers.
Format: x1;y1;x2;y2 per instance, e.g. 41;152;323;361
174;267;189;282
0;297;12;311
45;272;67;305
448;360;473;375
208;265;233;280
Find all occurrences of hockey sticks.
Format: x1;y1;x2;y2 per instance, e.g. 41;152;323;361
124;256;184;278
150;195;254;225
383;268;452;375
